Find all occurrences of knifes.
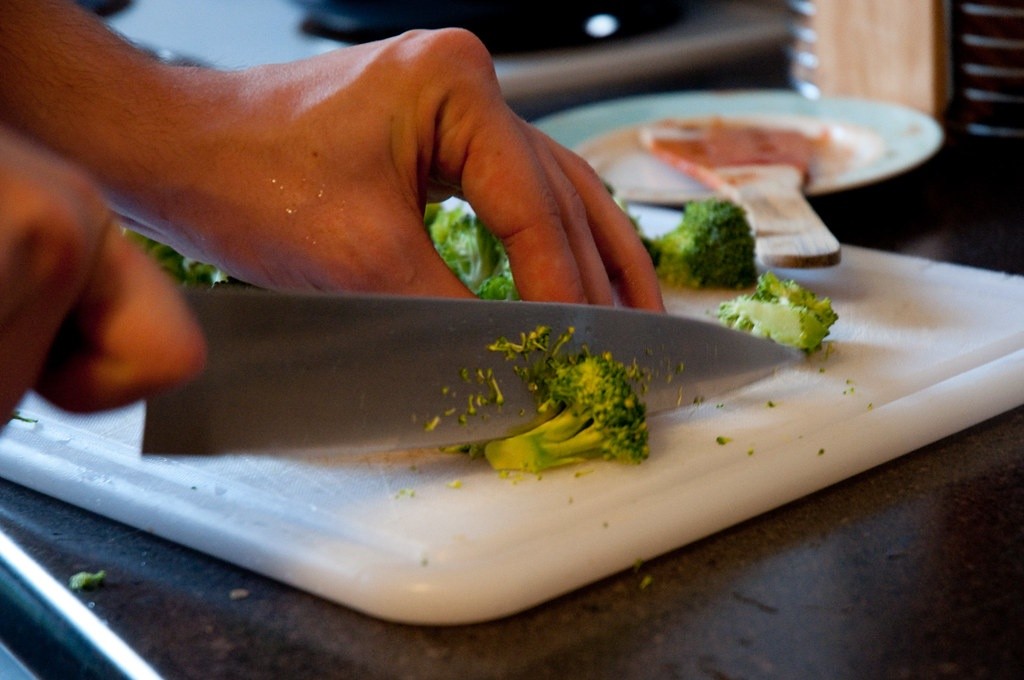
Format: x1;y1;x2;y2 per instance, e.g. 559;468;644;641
46;286;802;452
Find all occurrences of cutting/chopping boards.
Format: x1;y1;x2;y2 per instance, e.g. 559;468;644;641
0;196;1024;624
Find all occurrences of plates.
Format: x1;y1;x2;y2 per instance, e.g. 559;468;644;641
532;89;944;204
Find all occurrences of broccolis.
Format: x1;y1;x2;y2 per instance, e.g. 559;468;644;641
129;197;839;472
